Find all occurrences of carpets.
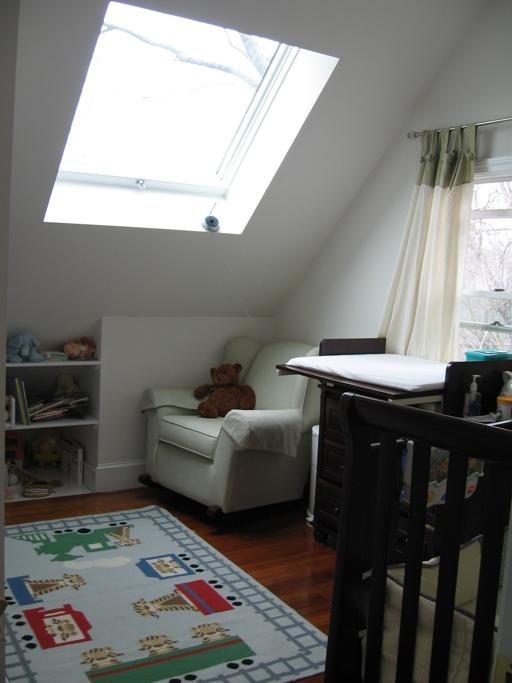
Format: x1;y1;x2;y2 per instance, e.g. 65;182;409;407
2;503;334;683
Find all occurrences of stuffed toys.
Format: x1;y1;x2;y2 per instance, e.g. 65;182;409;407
63;334;95;360
6;333;51;364
194;362;256;418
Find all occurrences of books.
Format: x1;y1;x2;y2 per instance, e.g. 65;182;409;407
23;480;56;497
63;440;83;487
7;377;92;422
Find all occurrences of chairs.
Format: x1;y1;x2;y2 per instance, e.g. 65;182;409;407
323;394;512;682
444;359;512;417
138;336;323;524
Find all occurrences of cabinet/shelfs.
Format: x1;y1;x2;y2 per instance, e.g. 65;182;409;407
4;360;99;505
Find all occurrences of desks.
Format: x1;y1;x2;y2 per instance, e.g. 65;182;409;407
274;337;447;567
